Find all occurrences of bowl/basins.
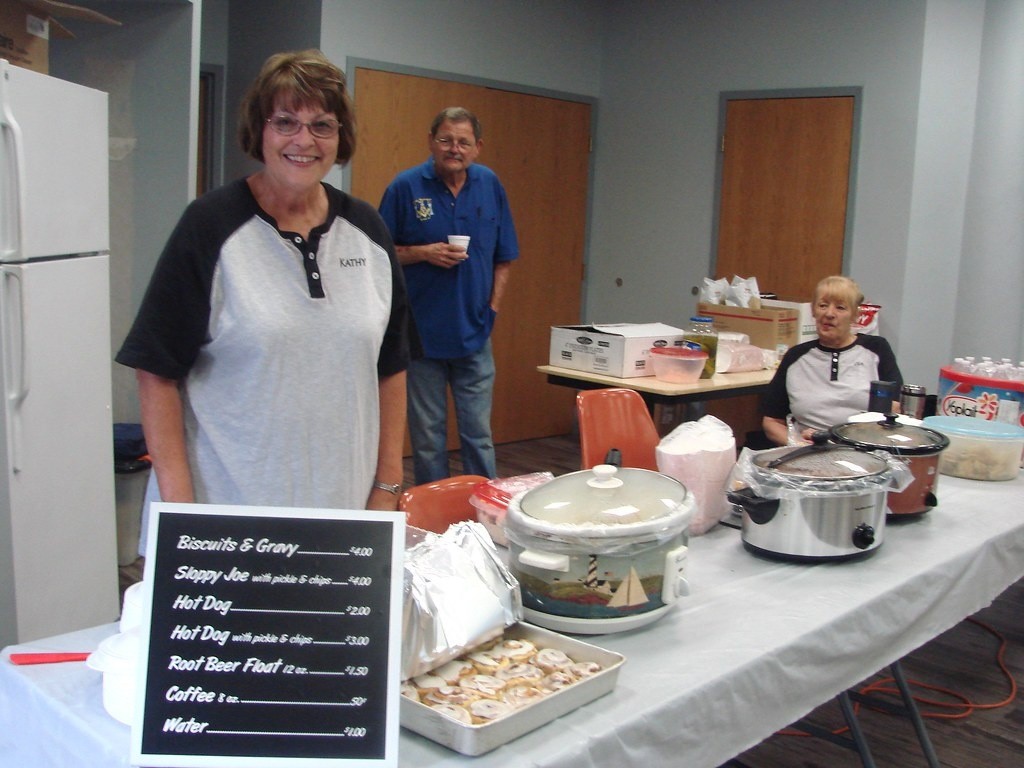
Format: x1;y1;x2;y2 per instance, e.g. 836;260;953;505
650;347;709;384
920;415;1024;481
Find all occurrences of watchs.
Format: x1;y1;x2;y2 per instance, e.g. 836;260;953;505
374;481;404;495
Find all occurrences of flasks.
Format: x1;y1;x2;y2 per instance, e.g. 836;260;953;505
867;380;894;414
900;384;926;420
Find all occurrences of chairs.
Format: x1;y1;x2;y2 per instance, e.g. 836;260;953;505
574;386;660;470
398;476;486;531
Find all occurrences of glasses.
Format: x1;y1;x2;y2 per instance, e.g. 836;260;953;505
265;115;344;138
435;138;477;152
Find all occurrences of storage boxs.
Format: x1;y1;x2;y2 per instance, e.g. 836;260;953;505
922;417;1024;482
931;367;1024;426
548;322;686;380
696;301;799;360
398;621;623;757
0;0;122;73
760;298;819;344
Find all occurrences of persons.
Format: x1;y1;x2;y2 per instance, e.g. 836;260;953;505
116;47;425;561
763;274;905;447
378;107;521;488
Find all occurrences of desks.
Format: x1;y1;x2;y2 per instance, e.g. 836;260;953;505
536;365;777;423
0;466;1024;768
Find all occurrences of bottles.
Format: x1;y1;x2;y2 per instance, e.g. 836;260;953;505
950;356;1024;382
682;316;718;380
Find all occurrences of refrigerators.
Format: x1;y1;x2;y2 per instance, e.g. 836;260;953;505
0;56;120;653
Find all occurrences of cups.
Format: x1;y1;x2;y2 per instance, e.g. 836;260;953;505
447;234;470;262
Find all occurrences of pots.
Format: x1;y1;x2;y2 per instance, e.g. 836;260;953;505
506;463;697;635
828;413;950;518
727;430;892;566
